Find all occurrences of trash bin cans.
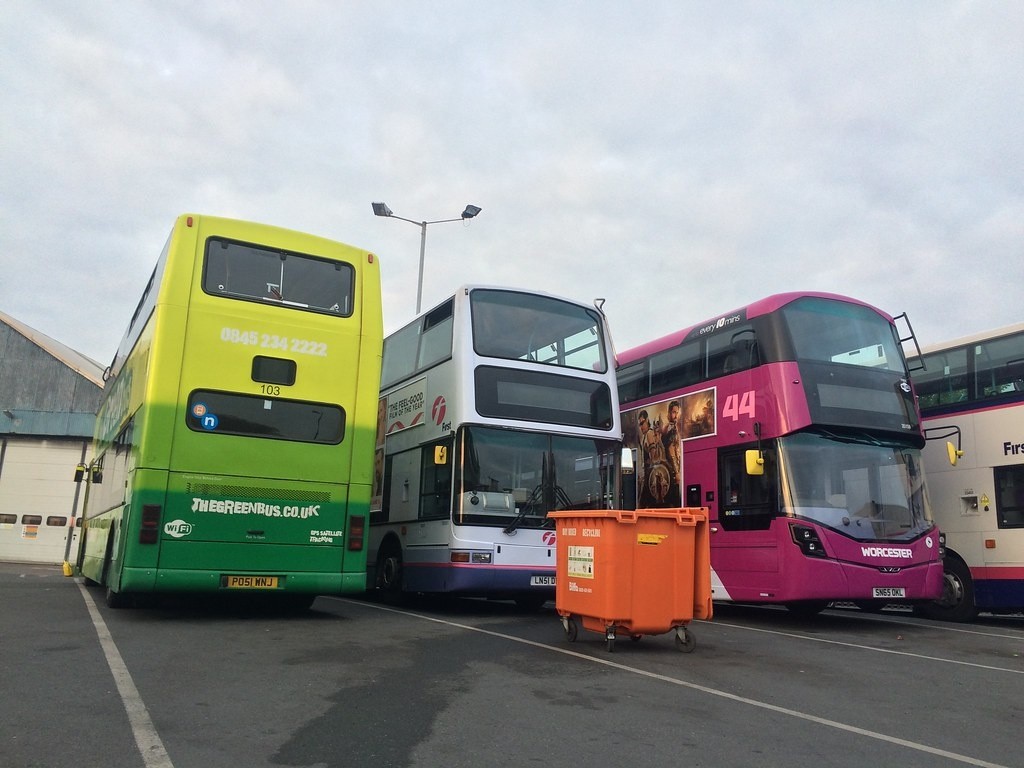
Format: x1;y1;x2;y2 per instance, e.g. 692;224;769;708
546;506;714;655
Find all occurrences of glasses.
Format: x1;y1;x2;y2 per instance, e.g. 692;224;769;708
639;419;646;426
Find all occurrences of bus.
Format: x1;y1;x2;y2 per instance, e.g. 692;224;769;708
904;322;1024;621
76;214;383;613
376;286;625;609
612;289;962;615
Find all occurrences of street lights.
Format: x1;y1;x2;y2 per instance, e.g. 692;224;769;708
372;201;482;316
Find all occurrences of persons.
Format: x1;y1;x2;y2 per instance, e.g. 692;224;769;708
639;401;680;508
